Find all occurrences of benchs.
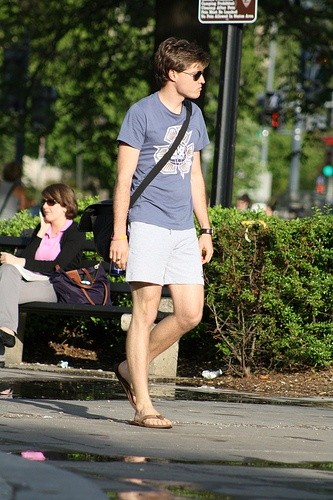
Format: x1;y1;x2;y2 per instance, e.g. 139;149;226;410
0;234;179;376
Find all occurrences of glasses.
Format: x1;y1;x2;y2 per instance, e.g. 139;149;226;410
41;198;57;205
183;71;207;81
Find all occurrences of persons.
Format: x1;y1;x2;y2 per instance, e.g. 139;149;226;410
236;194;273;219
0;162;26;220
0;184;87;368
108;35;214;429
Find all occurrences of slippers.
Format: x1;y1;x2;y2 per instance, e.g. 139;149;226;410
113;361;136;410
129;414;172;429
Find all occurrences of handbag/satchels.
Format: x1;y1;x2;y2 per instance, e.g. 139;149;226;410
79;196;128;264
51;263;112;306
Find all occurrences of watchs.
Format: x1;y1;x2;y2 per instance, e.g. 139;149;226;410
199;228;213;236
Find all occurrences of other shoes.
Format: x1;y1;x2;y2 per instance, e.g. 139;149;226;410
0;330;16;348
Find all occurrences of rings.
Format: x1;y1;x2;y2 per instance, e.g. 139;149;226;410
116;256;121;261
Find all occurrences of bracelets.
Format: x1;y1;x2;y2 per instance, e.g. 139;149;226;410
113;235;127;240
